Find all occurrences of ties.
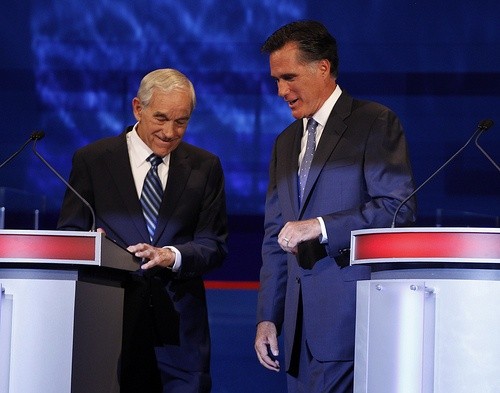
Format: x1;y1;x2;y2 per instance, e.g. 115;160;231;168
139;153;164;243
297;118;318;210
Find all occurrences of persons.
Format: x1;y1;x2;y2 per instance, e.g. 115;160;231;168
253;20;415;393
56;69;232;393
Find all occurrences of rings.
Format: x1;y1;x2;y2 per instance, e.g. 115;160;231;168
283;238;289;242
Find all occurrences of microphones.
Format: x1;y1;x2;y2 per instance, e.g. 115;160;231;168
475;120;500;172
33;129;95;232
391;117;489;229
0;129;38;167
